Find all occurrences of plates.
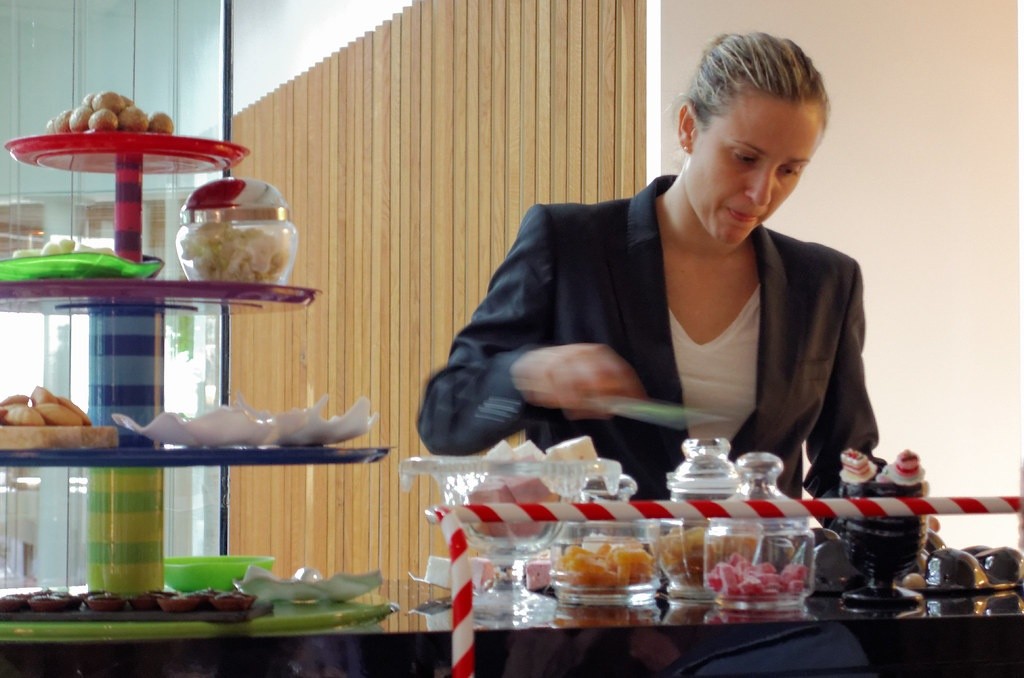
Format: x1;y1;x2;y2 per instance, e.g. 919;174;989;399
110;393;383;448
0;603;272;623
1;254;160;284
236;564;382;605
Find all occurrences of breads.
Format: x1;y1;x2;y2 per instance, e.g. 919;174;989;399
0;386;92;428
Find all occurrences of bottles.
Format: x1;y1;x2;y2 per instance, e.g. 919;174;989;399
175;177;297;284
550;438;815;609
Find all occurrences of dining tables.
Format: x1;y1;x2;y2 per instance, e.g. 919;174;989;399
0;575;1023;678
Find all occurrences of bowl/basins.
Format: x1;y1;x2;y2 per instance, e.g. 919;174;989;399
161;555;276;592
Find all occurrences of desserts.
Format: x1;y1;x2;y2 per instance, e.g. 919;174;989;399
0;589;256;614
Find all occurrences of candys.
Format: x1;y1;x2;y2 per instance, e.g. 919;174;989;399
560;543;807;600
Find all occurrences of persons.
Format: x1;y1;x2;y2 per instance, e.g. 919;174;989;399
415;31;878;499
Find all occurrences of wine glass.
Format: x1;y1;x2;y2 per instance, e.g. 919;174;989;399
837;481;928;612
